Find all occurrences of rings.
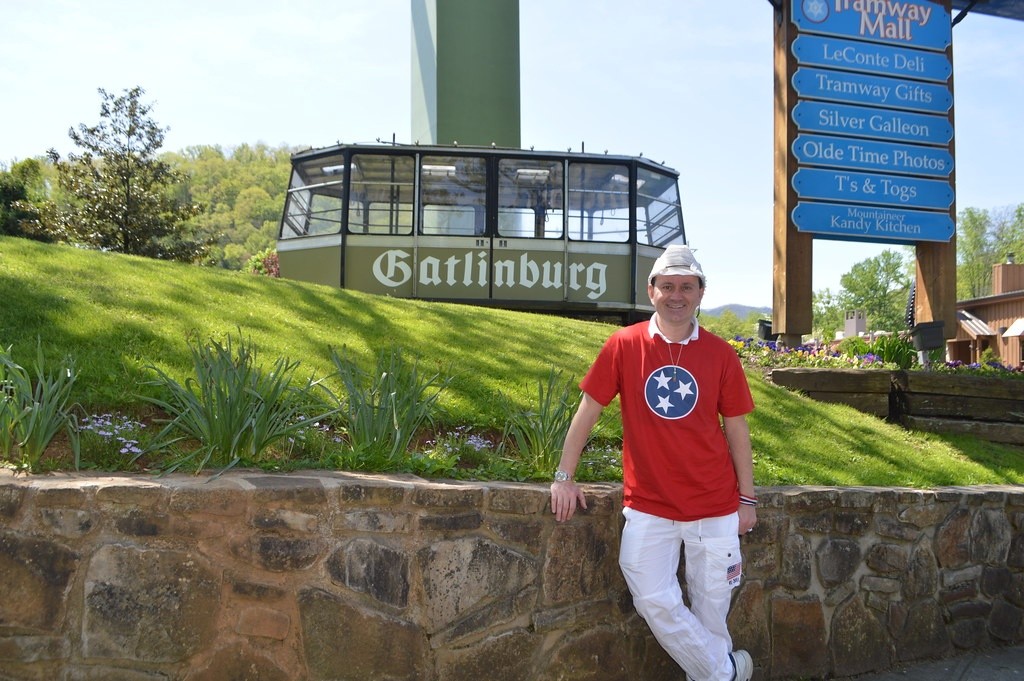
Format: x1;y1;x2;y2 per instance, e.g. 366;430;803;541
748;528;752;532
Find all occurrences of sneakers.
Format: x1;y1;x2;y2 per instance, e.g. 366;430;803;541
729;649;754;681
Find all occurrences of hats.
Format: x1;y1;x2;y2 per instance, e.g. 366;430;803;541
648;244;705;285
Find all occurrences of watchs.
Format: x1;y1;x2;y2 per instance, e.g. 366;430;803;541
554;470;572;481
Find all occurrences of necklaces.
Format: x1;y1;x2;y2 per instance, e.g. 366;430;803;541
669;343;683;382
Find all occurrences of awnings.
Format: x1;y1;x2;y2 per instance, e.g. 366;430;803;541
1002;319;1024;337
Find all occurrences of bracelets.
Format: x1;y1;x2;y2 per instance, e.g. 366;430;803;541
740;494;757;506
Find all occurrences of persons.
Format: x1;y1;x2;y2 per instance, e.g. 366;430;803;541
550;245;755;681
